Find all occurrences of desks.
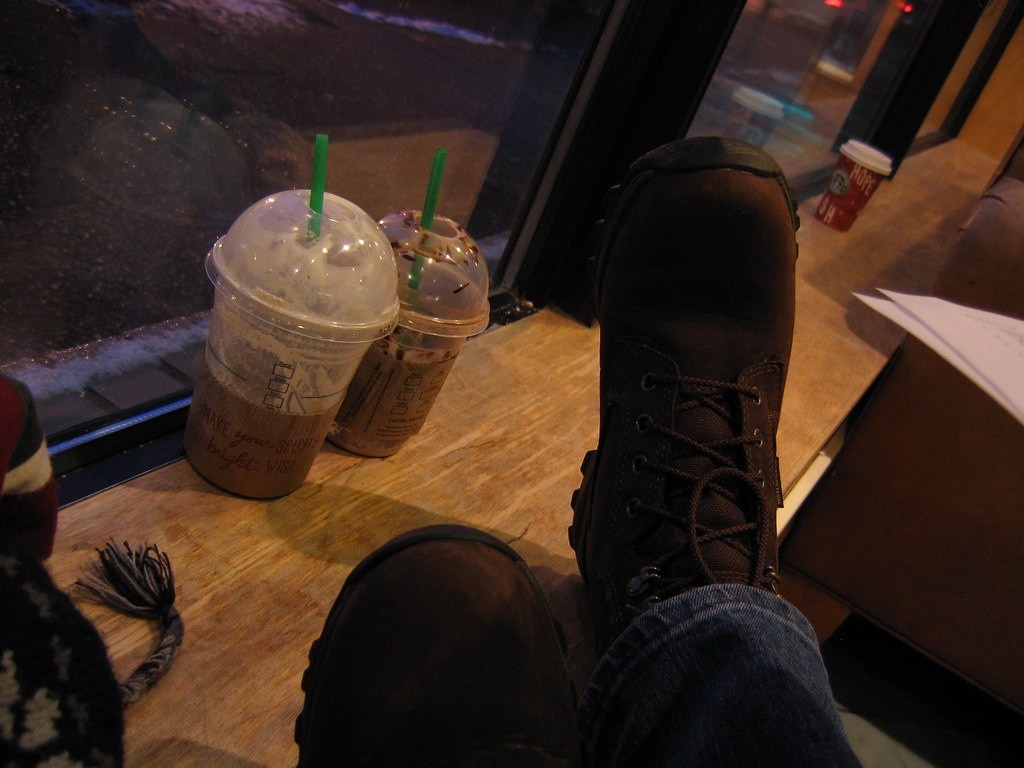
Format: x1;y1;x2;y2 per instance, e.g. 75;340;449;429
38;133;1000;766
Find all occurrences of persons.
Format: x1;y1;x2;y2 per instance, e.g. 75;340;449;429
293;137;867;768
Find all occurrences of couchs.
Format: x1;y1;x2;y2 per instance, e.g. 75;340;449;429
772;141;1023;724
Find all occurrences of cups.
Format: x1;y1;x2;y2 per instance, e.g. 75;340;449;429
324;208;491;459
813;137;893;233
181;188;402;501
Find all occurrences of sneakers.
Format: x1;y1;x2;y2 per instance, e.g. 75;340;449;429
568;137;798;658
293;522;580;768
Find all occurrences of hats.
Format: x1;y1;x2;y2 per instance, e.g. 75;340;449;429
0;539;185;768
